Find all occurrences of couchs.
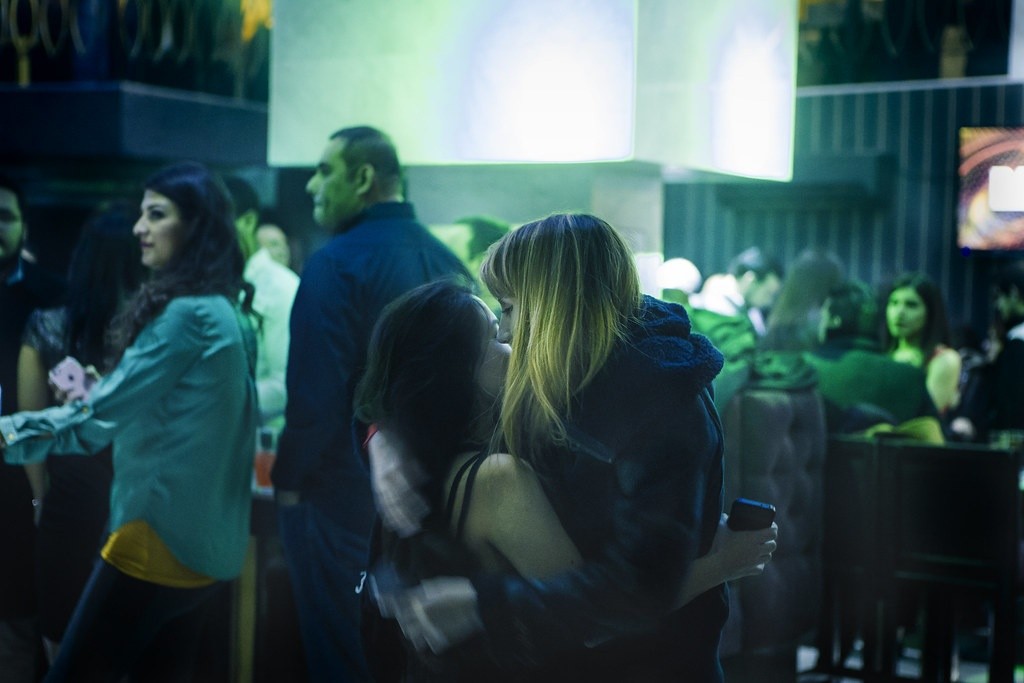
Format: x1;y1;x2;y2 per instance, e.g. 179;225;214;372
717;389;827;683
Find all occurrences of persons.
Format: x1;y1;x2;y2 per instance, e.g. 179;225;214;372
352;212;729;683
0;163;301;681
270;126;472;683
361;277;778;683
649;248;1024;683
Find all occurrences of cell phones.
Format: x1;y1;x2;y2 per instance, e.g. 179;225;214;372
49;355;96;402
727;498;776;530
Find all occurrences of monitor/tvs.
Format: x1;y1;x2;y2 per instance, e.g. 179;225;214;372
953;122;1024;254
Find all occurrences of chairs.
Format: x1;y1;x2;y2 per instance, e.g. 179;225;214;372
814;431;1024;683
